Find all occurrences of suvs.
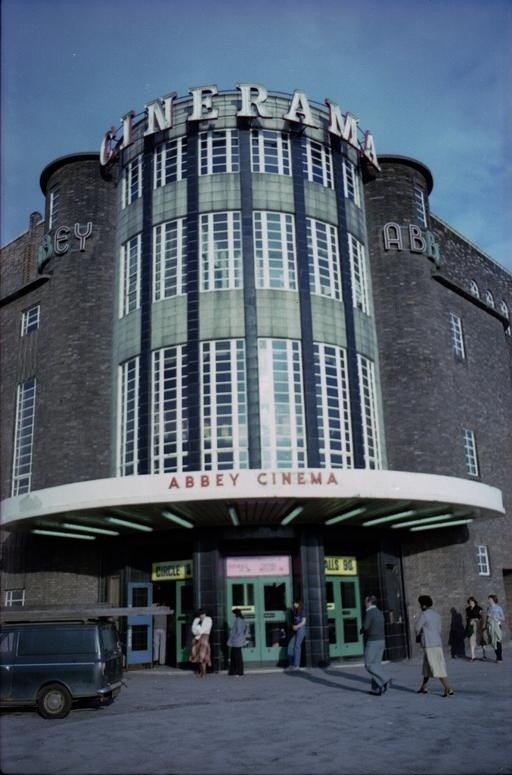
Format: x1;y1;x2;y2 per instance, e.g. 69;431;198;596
3;622;127;719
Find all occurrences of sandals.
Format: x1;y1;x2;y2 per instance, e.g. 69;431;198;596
417;688;454;697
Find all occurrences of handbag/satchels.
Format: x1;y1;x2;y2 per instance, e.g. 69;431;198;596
416;634;422;643
464;624;474;638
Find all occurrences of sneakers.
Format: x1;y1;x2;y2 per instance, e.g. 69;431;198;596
469;656;504;664
371;677;392;696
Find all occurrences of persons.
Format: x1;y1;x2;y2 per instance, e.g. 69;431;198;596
484;594;505;663
464;596;488;663
152;601;170;666
189;607;213;679
358;593;394;697
413;594;454;698
286;598;307;672
226;607;250;677
